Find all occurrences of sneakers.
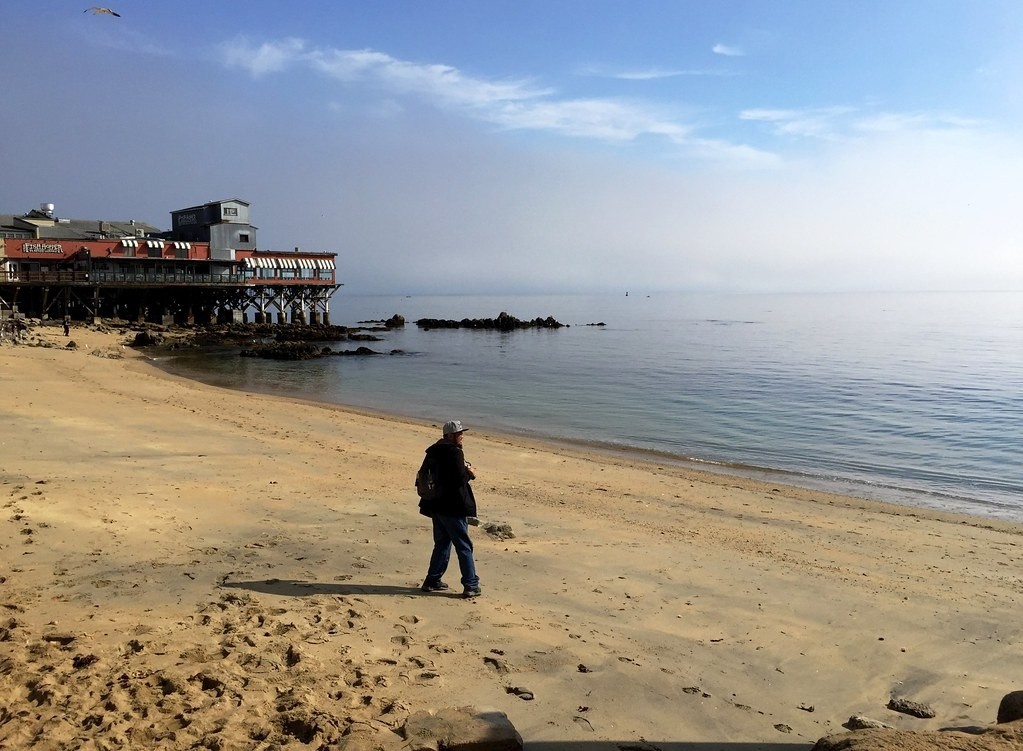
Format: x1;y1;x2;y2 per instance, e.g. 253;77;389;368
421;582;449;592
463;587;482;597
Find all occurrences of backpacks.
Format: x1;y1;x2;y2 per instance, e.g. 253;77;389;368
415;456;443;500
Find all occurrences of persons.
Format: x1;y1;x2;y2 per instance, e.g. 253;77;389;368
418;419;485;597
64;319;70;336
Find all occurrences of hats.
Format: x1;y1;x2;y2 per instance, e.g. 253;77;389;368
442;419;469;436
